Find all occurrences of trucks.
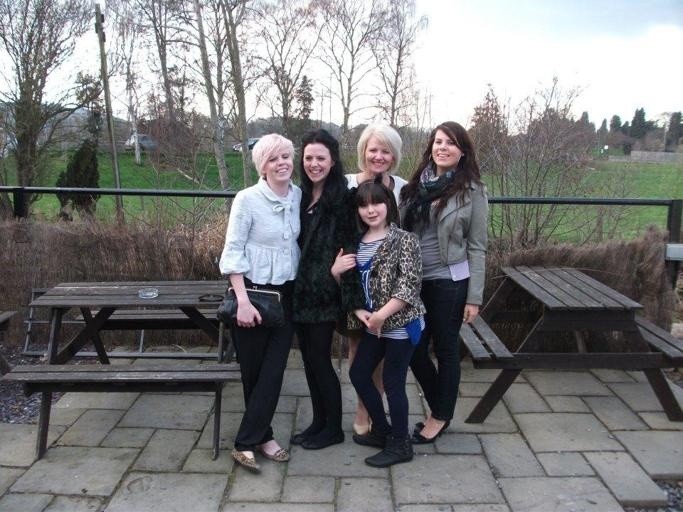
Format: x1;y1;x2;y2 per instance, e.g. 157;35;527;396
0;127;18;159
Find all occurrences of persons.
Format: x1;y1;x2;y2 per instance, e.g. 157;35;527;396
331;180;427;467
290;128;380;451
396;121;488;443
217;132;301;472
342;123;409;437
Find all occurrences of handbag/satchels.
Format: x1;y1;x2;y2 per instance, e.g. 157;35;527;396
216;286;286;329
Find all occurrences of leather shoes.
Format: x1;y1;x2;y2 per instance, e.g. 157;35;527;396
353;420;373;435
290;430;345;449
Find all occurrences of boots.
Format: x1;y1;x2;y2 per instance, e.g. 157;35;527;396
366;433;414;468
353;424;390;449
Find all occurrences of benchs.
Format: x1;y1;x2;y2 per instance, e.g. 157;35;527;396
73;307;222;334
631;312;683;364
459;307;515;369
0;362;243;394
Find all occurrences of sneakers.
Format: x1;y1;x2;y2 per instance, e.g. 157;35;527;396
231;448;259;471
257;444;290;461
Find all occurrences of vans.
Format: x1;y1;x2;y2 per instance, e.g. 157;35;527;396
123;132;156;152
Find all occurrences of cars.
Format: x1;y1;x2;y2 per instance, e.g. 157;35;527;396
232;136;259;151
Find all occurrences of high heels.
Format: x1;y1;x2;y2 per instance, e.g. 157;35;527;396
411;419;450;444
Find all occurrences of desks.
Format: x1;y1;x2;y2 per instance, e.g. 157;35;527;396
25;279;236;462
462;264;682;424
